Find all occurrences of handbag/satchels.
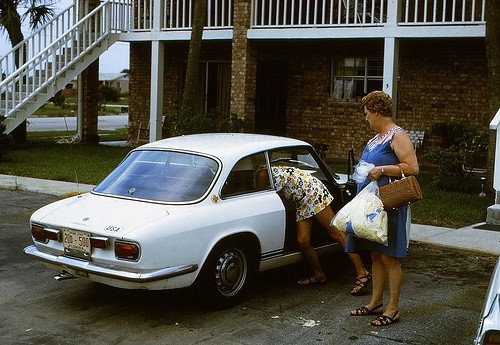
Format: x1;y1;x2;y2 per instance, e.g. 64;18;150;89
376;164;423;212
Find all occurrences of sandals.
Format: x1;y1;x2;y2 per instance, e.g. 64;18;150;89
298;274;328;286
350;303;384;316
350;272;372;294
370;310;399;326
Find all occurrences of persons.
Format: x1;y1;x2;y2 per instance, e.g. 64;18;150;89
345;91;419;326
253;165;372;296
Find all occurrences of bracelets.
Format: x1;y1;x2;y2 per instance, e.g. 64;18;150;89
380;166;385;175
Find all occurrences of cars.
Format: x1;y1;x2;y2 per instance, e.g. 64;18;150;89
22;132;359;307
